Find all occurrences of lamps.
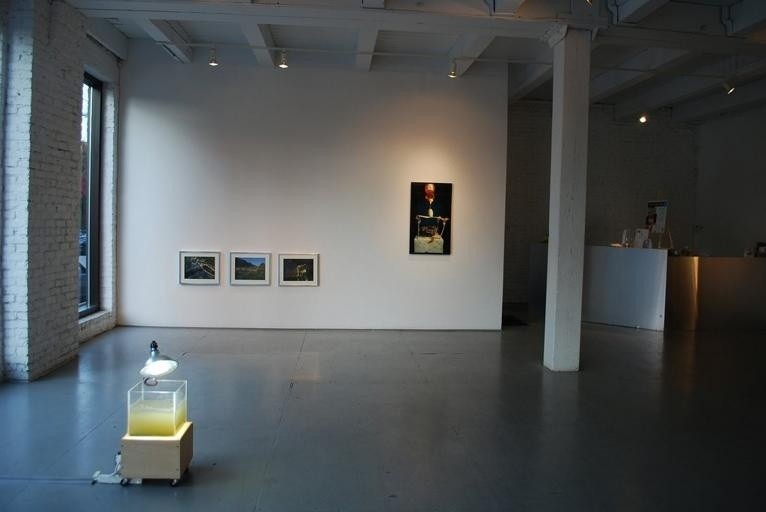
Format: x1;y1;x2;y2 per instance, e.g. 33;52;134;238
639;110;649;125
204;48;461;84
140;340;177;382
720;78;737;95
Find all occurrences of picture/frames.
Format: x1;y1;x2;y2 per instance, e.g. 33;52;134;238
178;250;320;288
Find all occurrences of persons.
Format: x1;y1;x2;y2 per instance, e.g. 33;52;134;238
418;183;441;237
295;259;308;281
